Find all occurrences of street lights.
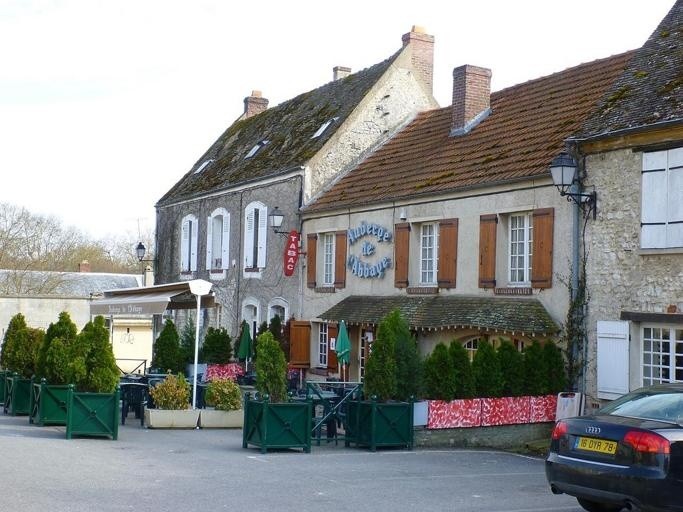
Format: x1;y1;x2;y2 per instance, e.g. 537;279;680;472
185;278;212;410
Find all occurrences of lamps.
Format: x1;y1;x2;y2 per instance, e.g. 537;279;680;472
136;242;158;263
547;145;597;219
399;206;414;234
266;203;299;237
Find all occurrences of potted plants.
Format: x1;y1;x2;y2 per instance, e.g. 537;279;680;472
5;326;44;416
0;315;26;405
69;314;122;444
343;310;412;452
239;330;315;456
26;308;76;429
197;376;243;429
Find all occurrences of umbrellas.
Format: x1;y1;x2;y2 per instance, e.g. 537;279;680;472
238;320;253;373
335;319;351;387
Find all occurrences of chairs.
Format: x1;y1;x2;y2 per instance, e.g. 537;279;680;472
270;379;370;450
114;368;206;427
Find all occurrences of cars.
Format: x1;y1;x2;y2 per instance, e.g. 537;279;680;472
544;384;683;512
